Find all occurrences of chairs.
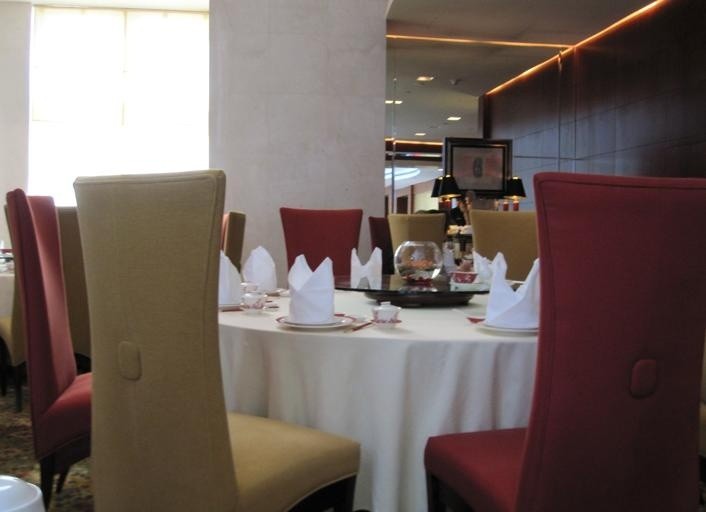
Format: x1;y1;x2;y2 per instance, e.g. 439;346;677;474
1;204;28;413
367;202;541;281
278;207;365;283
219;209;247;273
423;178;705;510
72;177;361;510
5;190;92;512
57;204;92;362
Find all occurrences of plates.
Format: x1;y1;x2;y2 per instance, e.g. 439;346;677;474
476;320;538;336
240;307;266;314
276;311;357;330
368;318;402;328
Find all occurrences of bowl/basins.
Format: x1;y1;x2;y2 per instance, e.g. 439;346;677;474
448;269;479;284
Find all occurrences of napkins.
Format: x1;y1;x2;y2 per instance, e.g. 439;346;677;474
218;249;244;307
349;245;383;290
486;253;540;329
241;246;277;291
286;254;336;324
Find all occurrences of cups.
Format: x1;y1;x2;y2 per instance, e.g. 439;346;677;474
242;292;267;308
371;300;401;323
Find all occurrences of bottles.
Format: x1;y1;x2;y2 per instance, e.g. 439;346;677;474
503;198;509;210
513;196;519;211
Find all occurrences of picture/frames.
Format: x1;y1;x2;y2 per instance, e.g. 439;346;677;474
444;137;512;197
